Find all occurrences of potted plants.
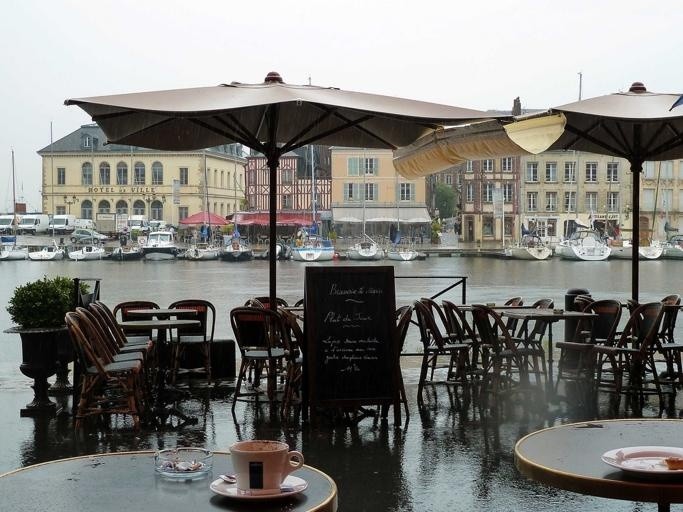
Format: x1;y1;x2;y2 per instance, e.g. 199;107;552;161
3;271;67;417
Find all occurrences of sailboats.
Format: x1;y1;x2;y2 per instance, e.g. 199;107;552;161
180;144;338;264
387;165;420;262
344;147;386;262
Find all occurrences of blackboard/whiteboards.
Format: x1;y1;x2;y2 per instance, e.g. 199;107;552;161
304;266;400;405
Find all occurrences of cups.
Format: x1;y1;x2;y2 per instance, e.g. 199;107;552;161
227;438;306;491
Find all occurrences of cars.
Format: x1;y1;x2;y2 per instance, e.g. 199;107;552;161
69;228;107;246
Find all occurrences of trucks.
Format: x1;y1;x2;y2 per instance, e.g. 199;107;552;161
0;212;171;236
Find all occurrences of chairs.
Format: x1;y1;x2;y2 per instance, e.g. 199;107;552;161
57;297;304;432
396;292;683;409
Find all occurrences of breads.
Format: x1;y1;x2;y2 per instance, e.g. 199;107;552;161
665;457;683;470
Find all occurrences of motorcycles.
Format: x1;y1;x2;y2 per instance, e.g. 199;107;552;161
118;232;128;246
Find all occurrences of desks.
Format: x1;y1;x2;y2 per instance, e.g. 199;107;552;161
1;447;339;512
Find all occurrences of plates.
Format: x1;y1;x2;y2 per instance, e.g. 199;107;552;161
210;475;309;500
601;445;683;479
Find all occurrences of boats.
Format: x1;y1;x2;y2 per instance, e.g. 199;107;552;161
509;226;683;261
141;227;177;261
0;245;146;261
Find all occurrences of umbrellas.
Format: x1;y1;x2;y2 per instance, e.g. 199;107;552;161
65;66;497;442
393;77;681;407
179;211;230;226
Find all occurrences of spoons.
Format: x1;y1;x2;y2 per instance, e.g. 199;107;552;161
218;474;235;485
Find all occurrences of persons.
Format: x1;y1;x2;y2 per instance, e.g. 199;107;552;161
169;225;174;238
